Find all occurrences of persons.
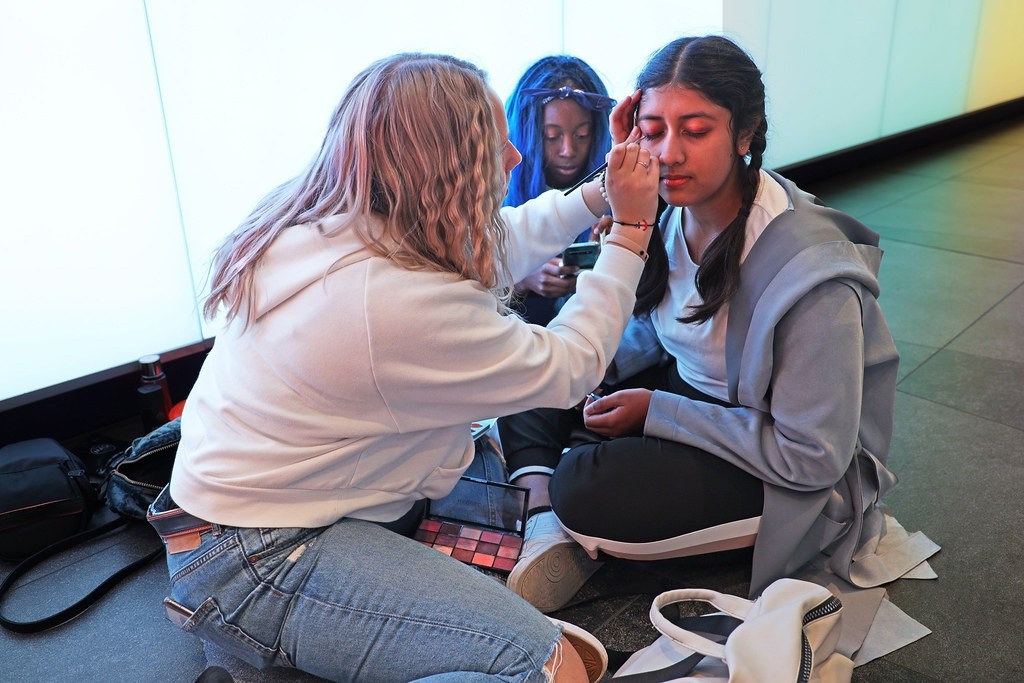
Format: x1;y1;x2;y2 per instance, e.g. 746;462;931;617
496;34;941;661
499;52;618;325
145;51;660;683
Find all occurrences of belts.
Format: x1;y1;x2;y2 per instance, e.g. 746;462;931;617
145;511;212;535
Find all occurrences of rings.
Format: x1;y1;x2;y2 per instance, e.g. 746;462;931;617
636;161;649;169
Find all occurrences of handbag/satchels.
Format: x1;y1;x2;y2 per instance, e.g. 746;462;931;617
610;575;855;683
0;438;93;563
106;419;184;522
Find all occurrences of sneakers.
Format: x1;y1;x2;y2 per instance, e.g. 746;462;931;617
505;508;605;612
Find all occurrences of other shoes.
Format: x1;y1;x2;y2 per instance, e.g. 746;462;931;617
542;618;609;682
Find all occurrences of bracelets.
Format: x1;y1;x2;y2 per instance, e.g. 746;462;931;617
612;217;656;230
598;171;608;202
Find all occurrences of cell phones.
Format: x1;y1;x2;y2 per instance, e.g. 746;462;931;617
562;241;600;280
